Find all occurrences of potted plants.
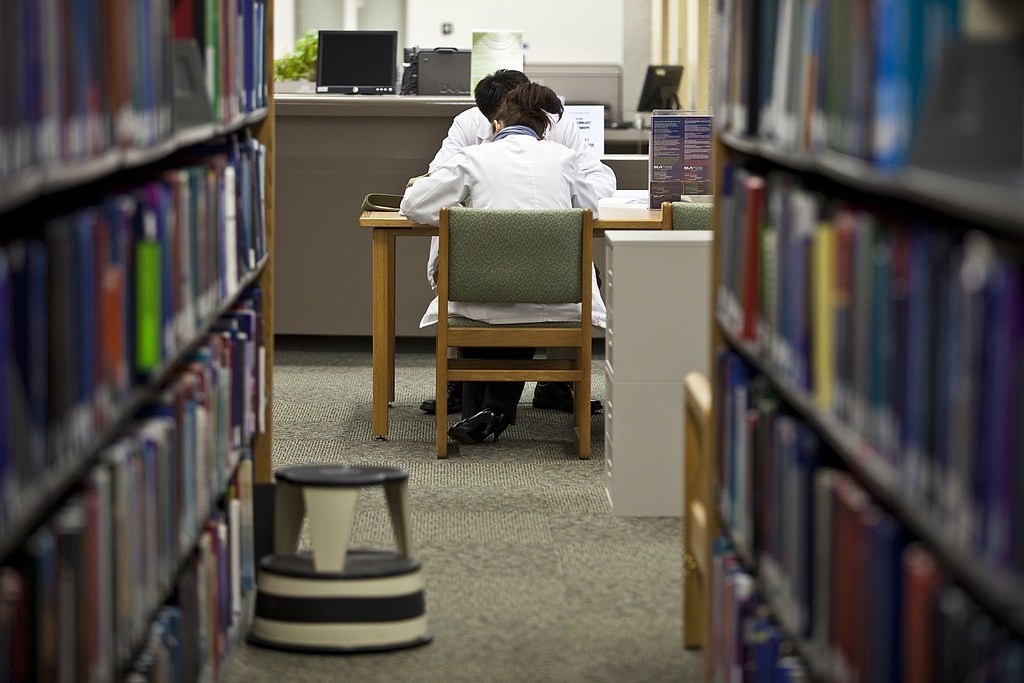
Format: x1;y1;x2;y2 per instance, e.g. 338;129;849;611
273;32;318;83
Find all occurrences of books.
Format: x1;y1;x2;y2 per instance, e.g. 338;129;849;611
705;0;1024;683
0;0;270;682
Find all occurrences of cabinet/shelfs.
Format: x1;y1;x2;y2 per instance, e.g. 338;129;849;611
603;228;714;521
696;125;1024;683
0;0;274;683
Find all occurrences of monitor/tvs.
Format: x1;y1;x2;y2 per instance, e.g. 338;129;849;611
636;65;684;110
315;31;398;95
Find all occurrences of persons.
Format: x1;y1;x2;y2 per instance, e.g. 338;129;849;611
400;83;607;442
419;69;616;412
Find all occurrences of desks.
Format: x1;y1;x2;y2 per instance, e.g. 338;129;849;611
360;208;661;439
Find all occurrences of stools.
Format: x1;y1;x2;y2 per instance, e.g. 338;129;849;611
243;464;436;657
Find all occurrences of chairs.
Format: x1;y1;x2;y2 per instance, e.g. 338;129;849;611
437;206;594;461
661;201;713;231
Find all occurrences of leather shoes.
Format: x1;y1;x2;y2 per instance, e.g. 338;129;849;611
532;381;603;414
421;381;462;414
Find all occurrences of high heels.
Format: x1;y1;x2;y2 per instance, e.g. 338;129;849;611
447;407;512;444
